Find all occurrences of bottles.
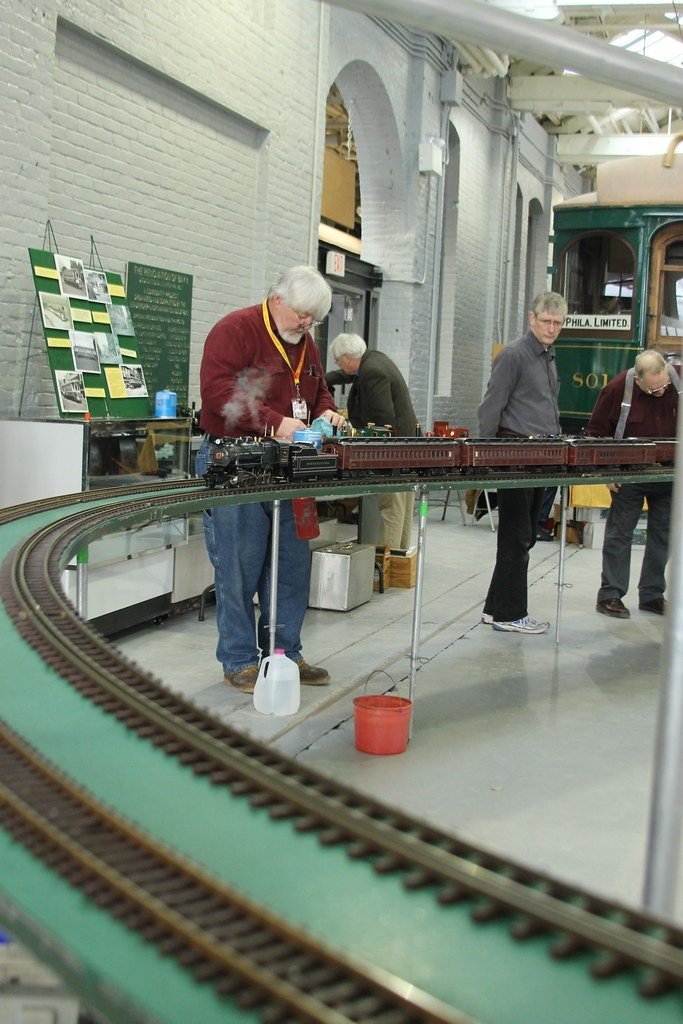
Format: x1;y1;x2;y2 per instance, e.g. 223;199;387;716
254;648;299;715
155;389;177;418
293;427;322;451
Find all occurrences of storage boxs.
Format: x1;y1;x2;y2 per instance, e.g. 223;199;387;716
556;519;586;544
308;542;416;612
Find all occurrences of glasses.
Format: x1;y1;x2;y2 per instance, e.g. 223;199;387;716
292;311;326;328
644;380;672;395
539;319;567;328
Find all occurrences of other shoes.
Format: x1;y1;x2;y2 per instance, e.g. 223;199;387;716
639;598;669;615
297;659;331;685
596;598;630;618
224;666;260;693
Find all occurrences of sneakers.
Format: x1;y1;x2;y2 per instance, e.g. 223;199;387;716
492;616;550;634
481;613;494;624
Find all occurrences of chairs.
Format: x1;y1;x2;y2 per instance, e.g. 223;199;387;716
415;426;472;526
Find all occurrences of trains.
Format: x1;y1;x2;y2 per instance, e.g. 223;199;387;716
201;425;680;488
543;152;683;439
46;305;67;321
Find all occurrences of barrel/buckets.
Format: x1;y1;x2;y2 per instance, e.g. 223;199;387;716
353;669;411;754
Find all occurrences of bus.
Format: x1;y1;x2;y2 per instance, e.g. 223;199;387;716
63;269;83;289
60;380;83;403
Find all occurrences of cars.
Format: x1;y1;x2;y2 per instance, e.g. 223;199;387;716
126;377;143;389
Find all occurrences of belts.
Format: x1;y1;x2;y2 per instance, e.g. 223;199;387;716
499;426;525;438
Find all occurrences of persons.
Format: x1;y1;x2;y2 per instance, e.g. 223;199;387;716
588;349;683;620
323;331;423;550
477;291;570;634
196;264;345;694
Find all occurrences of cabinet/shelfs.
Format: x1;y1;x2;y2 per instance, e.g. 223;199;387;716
0;419;215;634
